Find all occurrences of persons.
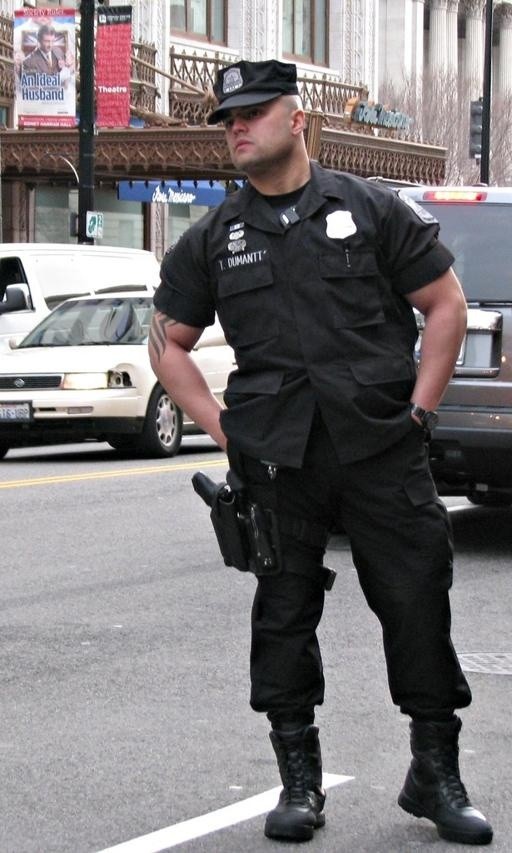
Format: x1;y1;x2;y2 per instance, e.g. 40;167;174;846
15;23;71;90
145;58;496;847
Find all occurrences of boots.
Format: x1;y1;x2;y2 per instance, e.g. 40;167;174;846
264;726;324;841
398;719;492;844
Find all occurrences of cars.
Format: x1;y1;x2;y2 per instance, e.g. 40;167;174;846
1;290;234;459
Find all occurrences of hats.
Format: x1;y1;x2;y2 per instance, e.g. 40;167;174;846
206;59;297;126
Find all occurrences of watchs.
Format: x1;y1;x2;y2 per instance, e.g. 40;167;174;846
410;402;440;432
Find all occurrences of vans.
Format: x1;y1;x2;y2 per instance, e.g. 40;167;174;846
363;186;512;507
0;244;161;353
